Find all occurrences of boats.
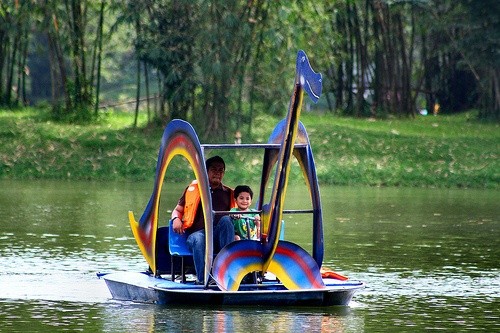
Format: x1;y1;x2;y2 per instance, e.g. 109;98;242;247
97;50;364;307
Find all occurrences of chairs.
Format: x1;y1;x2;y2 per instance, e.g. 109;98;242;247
168;220;196;285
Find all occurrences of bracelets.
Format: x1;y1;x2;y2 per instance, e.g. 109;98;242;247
170;217;180;223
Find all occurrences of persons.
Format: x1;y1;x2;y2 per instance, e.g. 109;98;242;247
229;185;262;241
170;156;238;286
